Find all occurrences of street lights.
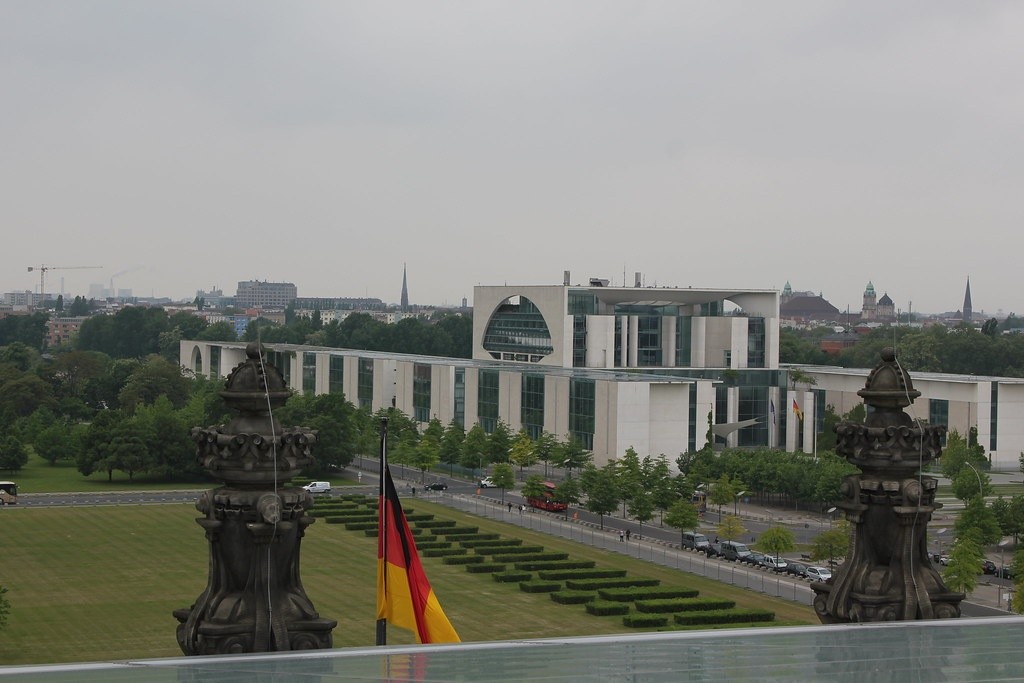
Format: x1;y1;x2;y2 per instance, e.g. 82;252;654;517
965;461;982;501
937;528;947;570
827;506;836;565
507;447;570;478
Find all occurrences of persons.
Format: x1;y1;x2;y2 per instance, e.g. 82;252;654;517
619;528;625;542
518;504;527;515
411;486;415;496
626;528;630;541
508;502;512;512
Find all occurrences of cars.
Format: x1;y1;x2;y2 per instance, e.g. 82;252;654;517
998;538;1009;588
995;566;1015;581
705;542;722;557
978;560;997;574
806;567;832;582
785;562;808;577
425;482;448;491
941;557;953;565
828;556;845;565
746;553;765;566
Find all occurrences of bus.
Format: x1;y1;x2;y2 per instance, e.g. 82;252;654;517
665;490;707;514
526;480;568;512
0;481;20;504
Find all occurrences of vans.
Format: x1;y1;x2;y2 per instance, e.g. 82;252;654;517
303;482;332;493
764;554;787;572
683;532;709;550
481;476;504;489
721;540;751;561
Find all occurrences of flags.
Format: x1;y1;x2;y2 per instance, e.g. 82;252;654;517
376;439;462;644
770;400;776;424
793;400;802;421
